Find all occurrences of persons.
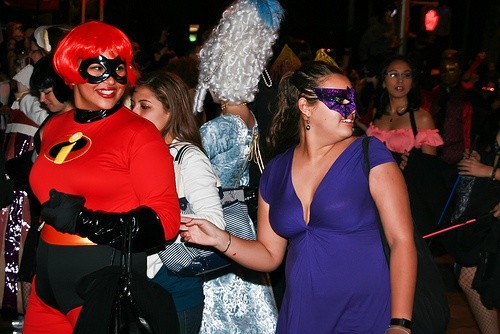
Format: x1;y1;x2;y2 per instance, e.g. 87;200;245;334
179;61;417;334
0;20;181;334
192;0;284;334
0;20;500;334
130;70;227;334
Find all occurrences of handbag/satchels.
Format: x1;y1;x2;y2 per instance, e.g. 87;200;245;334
159;145;255;276
73;221;180;334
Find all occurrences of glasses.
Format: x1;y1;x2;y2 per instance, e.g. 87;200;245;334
384;71;412;80
29;49;41;54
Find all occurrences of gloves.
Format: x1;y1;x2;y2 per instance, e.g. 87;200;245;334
41;190;166;253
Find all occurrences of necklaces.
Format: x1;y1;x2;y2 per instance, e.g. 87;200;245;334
388;105;406;129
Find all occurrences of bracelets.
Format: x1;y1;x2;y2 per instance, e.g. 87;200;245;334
491;166;498;180
389;318;412;328
385;327;409;334
222;229;231;253
